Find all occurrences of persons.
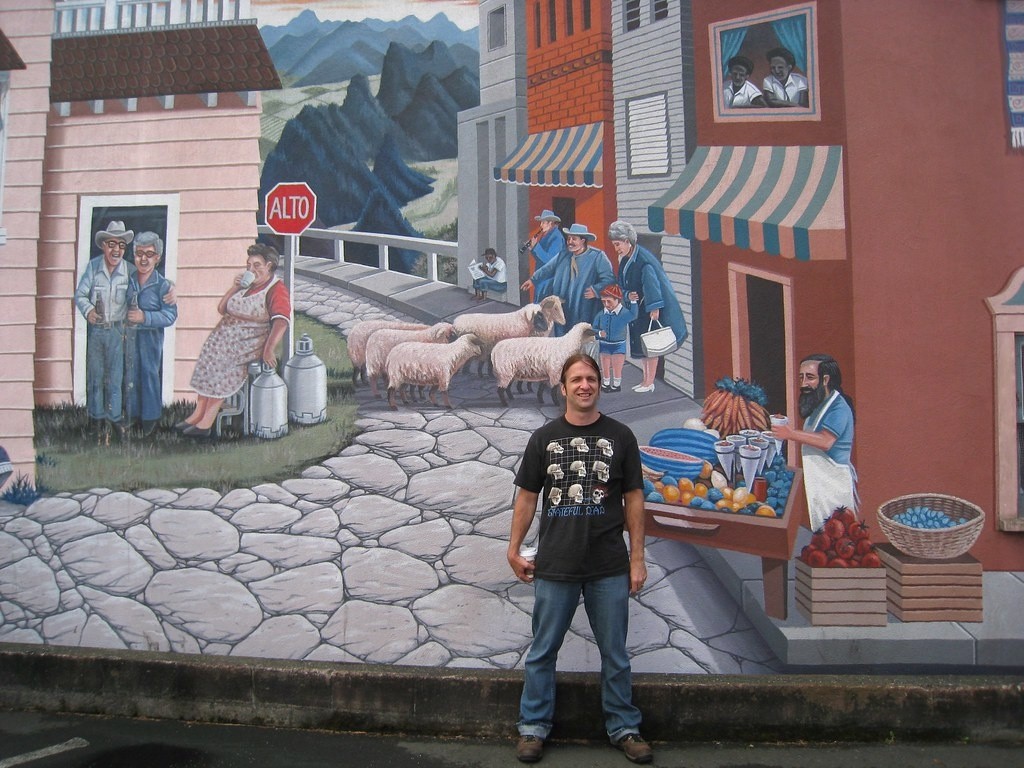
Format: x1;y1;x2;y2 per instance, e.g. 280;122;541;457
507;354;654;765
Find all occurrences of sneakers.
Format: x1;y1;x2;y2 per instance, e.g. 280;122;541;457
516;735;545;762
616;733;654;763
